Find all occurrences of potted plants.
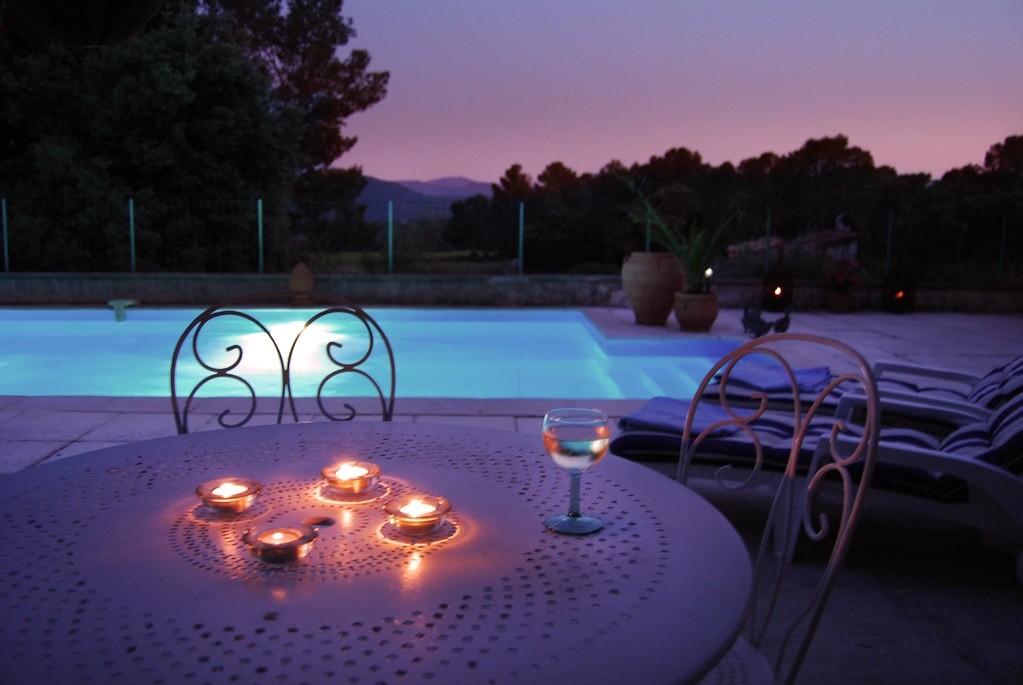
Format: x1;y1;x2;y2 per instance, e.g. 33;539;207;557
271;237;324;306
618;173;738;332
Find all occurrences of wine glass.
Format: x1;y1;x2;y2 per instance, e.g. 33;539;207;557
542;409;611;534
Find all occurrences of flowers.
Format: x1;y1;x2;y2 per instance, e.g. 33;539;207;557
827;257;866;293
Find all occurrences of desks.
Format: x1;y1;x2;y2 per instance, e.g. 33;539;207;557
0;416;753;684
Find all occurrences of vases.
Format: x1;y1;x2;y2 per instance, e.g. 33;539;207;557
622;252;685;327
827;286;866;312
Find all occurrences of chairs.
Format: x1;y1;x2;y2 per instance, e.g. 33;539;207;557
168;289;398;435
609;350;1023;546
680;330;882;684
